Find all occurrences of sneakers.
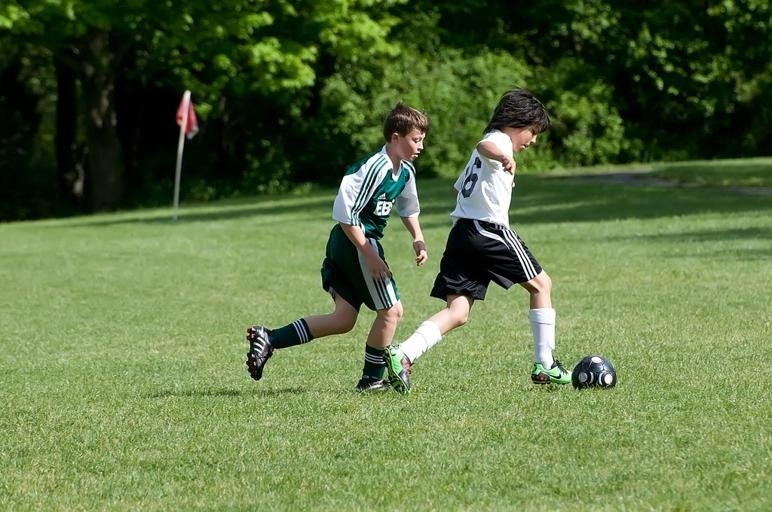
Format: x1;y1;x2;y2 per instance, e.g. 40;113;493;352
531;359;572;384
356;379;391;394
382;345;411;396
246;326;274;380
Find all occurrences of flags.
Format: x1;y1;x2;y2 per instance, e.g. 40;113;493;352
174;86;201;140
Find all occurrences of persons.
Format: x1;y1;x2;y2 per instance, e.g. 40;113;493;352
243;101;431;393
381;86;577;397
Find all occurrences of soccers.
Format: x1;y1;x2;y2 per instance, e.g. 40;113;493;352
572;354;616;393
